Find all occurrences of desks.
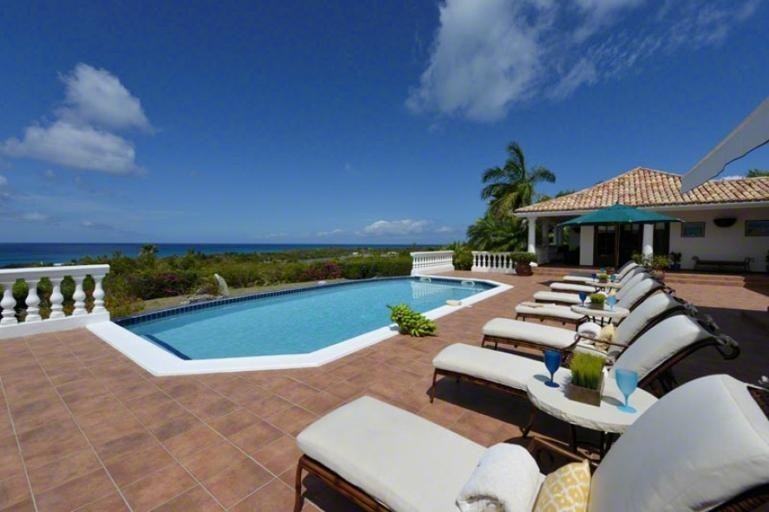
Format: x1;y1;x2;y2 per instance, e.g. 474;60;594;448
519;367;659;463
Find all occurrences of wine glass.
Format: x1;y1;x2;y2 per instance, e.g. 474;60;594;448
600;267;606;275
607;294;617;314
592;272;596;284
615;368;639;415
609;274;616;284
544;348;562;388
579;292;587;308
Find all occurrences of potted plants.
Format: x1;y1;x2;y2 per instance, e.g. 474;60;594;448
635;251;682;284
511;250;538;276
566;352;606;407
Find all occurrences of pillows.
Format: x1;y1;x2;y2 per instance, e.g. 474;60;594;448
533;459;591;511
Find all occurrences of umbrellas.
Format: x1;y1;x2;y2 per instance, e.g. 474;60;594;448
554;200;686;268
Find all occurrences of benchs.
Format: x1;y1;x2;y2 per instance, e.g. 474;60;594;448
691;256;751;275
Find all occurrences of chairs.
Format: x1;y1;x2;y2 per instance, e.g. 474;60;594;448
429;261;742;404
295;373;768;512
